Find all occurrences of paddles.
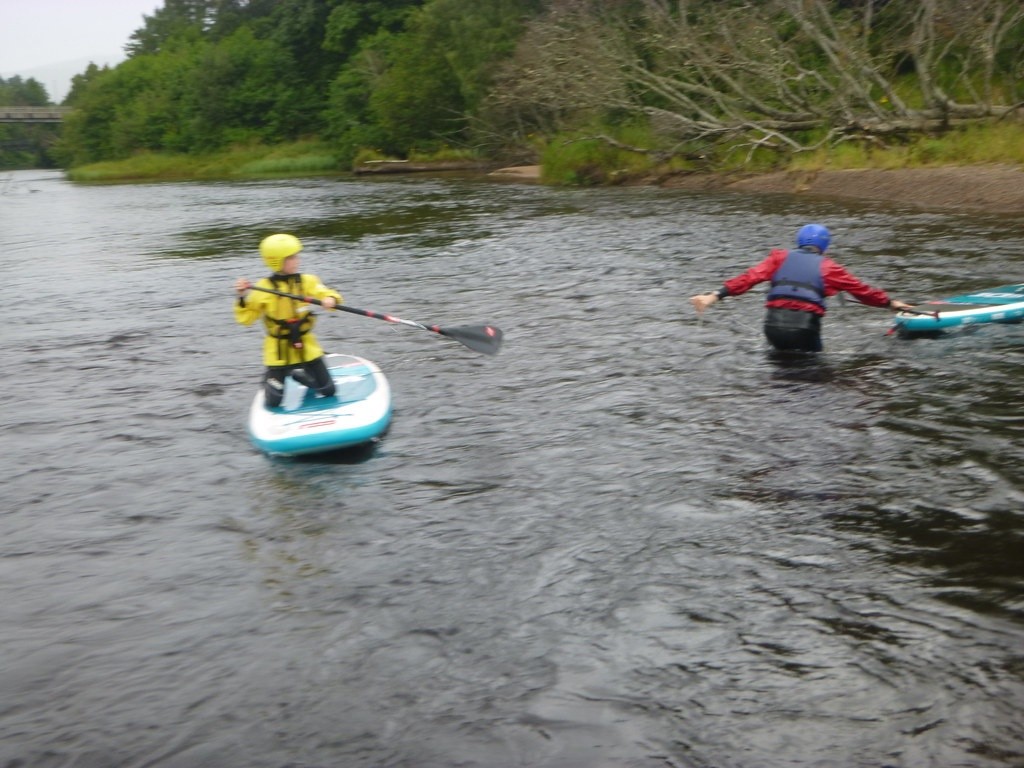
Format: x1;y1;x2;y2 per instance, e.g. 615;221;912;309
836;292;942;325
245;283;505;357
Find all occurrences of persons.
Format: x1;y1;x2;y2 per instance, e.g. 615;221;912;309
232;232;342;408
688;221;916;351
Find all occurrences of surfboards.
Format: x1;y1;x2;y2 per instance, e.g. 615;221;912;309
890;281;1024;333
245;351;394;460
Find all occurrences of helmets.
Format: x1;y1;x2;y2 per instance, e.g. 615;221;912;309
259;234;304;272
796;224;832;254
